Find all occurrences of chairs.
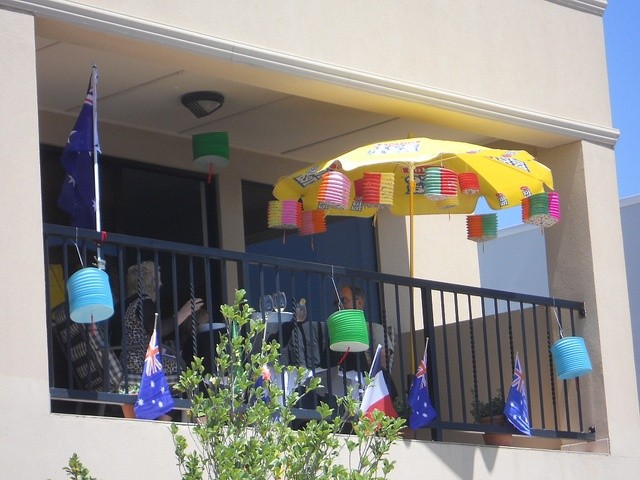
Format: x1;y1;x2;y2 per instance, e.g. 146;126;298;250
386;326;395;375
51;300;179;418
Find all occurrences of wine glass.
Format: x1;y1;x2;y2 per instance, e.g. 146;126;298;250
272;291;287;313
258;294;273;314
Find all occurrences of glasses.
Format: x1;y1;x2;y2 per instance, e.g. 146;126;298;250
334;296;359;303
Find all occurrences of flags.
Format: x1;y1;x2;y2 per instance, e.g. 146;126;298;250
249;362;280;428
359;344;400;438
502;355;532;437
56;63;102;232
133;324;174;420
406;346;438;431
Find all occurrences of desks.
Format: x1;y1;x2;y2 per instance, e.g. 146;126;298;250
191;312;293;407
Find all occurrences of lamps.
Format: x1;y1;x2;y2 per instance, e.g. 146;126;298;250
180;91;225;119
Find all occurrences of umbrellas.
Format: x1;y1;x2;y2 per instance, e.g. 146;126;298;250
272;132;555;375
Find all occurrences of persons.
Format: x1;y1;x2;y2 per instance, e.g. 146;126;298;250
117;260;205;421
333;284;396;376
182;310;210;365
